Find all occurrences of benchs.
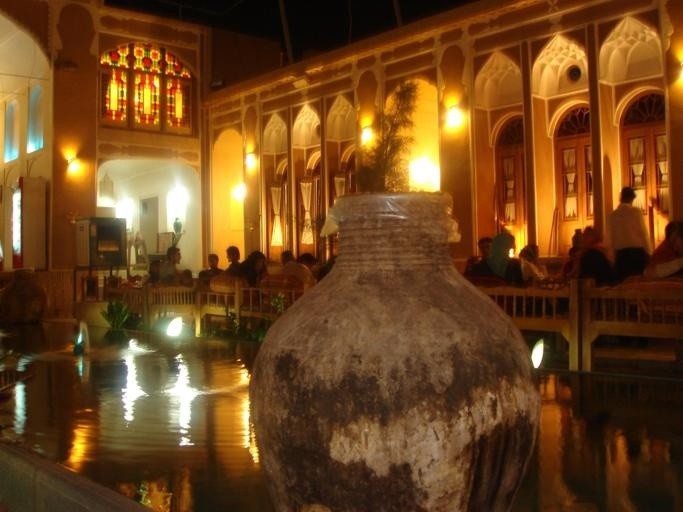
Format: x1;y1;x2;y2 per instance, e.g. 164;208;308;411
475;278;581;373
581;278;683;373
107;278;305;338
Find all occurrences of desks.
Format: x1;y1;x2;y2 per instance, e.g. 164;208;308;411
72;265;131;319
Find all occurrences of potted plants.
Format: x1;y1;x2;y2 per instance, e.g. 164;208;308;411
99;297;130;347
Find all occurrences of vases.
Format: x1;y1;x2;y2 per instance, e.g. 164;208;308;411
247;192;542;511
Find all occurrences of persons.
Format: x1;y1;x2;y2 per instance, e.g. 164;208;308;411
145;246;335;312
464;187;683;347
560;410;612;512
